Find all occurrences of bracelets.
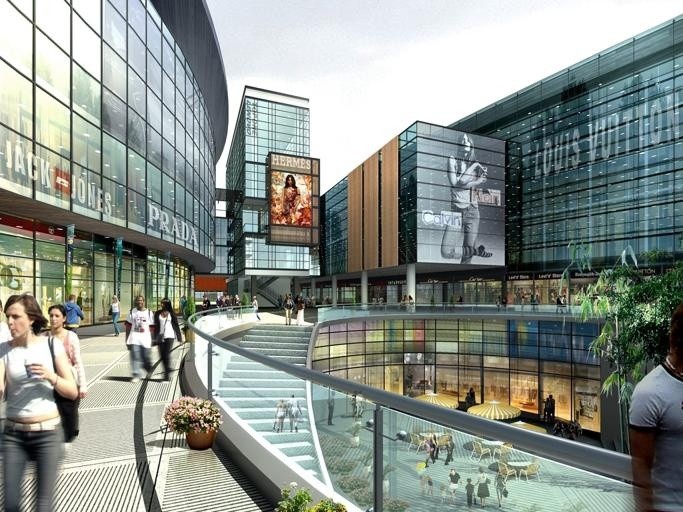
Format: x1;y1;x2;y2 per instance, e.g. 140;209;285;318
296;193;301;196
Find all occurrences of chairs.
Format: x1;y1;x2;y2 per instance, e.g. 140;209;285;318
469;439;541;483
408;432;451;458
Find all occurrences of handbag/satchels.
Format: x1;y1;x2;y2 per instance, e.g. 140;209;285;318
503;487;508;497
486;478;491;484
108;308;112;315
53;386;79;442
474;497;477;504
153;333;164;345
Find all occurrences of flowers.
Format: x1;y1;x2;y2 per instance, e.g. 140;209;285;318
164;395;223;434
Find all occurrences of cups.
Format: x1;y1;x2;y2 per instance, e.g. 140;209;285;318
24;363;40;380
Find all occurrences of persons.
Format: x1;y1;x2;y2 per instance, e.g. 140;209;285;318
280;174;301;224
627;304;683;512
326;388;336;426
0;291;84;512
415;430;507;510
496;287;571;315
370;293;466;313
271;397;288;434
252;295;260;320
440;133;493;264
540;393;599;442
277;292;334;326
110;293;241;384
287;394;302;433
460;387;477;412
351;396;364;419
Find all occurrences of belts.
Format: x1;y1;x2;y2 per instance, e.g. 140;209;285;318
5;415;62;432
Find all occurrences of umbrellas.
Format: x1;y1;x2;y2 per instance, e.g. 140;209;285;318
466;400;522;419
509;420;547;434
414;389;459;410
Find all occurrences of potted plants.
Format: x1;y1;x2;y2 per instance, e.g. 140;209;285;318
346;420;362;448
183;296;196;343
382;463;397;495
355;450;373;478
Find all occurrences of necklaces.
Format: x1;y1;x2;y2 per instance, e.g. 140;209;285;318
665;356;683;378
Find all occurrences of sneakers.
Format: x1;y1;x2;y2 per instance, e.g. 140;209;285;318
131;376;141;383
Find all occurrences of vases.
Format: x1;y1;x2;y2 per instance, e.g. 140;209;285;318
185;428;216;451
